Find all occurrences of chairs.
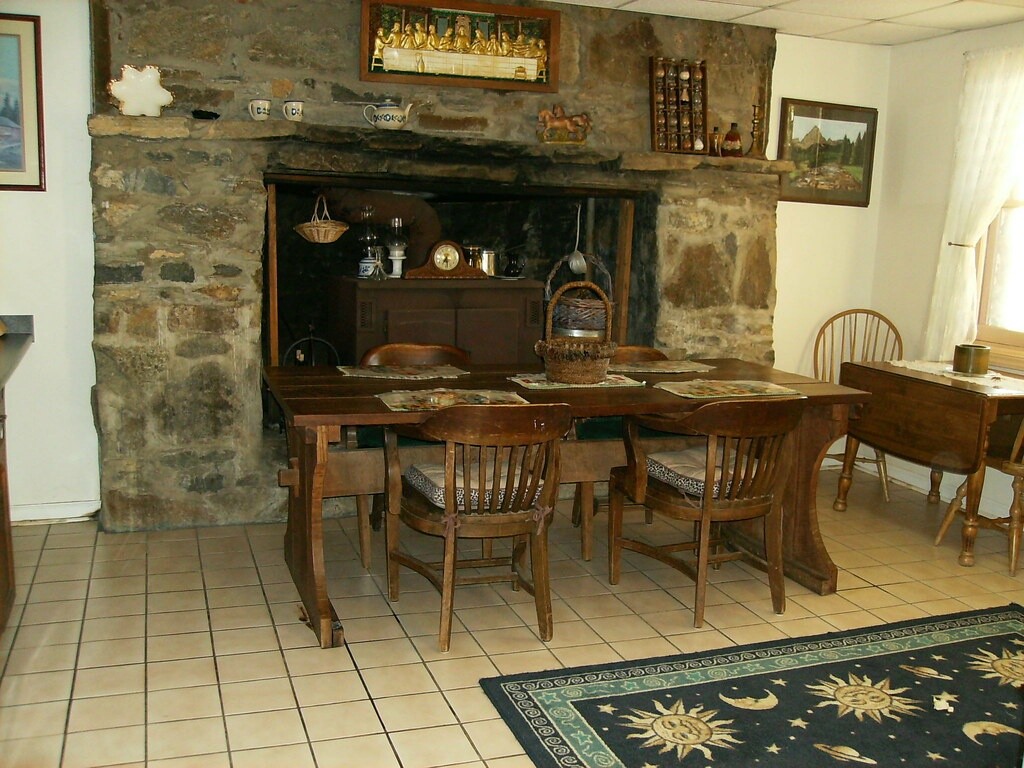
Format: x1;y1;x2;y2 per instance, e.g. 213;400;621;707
805;309;908;505
351;345;809;646
932;420;1024;577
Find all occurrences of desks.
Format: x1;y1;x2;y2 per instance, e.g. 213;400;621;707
257;353;871;648
836;358;1020;566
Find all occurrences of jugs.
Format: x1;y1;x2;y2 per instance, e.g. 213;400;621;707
506;251;526;277
461;245;482;270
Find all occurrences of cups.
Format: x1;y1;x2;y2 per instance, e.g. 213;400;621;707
482;251;496;276
248;99;271;120
282;100;305;122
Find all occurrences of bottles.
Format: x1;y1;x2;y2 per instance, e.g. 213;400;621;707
721;122;743;157
710;126;721;156
654;57;704;150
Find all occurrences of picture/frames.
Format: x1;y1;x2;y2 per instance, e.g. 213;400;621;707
0;12;46;193
780;98;877;210
360;0;560;93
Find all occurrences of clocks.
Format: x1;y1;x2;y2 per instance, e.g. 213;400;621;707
404;240;488;279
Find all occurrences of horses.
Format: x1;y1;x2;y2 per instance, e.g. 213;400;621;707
539;104;591;141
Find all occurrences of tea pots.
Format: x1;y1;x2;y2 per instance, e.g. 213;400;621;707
364;99;413;128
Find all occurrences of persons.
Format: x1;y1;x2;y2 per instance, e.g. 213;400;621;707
374;23;547;80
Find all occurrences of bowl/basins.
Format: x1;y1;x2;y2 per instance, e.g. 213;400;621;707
360;258;377;278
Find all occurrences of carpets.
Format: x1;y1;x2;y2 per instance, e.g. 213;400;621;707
483;604;1023;767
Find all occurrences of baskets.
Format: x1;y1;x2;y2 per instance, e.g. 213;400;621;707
293;194;349;243
534;282;617;383
544;252;615;328
